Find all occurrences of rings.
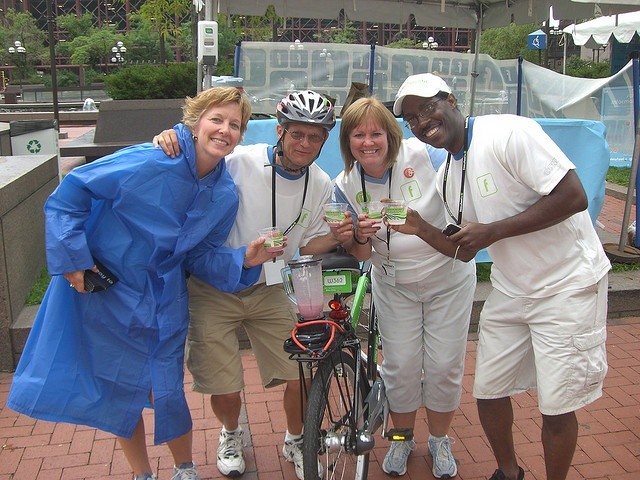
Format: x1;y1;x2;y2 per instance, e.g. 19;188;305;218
68;283;74;288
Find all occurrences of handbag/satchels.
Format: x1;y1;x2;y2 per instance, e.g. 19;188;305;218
84;257;118;293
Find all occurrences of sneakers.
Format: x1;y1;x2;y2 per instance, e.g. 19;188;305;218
216;425;246;477
487;465;524;480
427;436;458;478
382;436;417;476
173;465;199;479
283;435;324;480
133;473;158;480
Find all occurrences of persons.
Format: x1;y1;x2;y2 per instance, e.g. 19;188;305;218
154;89;353;480
6;87;287;480
332;95;479;479
381;73;614;480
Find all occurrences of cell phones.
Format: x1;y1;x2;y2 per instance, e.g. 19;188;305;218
440;223;462;235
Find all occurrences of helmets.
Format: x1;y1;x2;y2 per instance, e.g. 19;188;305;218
277;90;337;130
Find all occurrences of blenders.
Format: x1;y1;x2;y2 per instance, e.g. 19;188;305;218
280;254;331;345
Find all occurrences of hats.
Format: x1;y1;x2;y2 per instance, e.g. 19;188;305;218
393;72;452;117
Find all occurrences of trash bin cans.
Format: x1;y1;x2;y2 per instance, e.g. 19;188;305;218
9;118;62;182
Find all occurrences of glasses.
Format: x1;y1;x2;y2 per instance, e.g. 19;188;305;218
405;96;446;130
284;127;326;142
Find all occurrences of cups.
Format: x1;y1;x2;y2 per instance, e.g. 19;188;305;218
384;203;407;226
323;203;349;228
364;201;383;227
258;227;284;253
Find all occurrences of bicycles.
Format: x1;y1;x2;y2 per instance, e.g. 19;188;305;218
283;245;415;479
